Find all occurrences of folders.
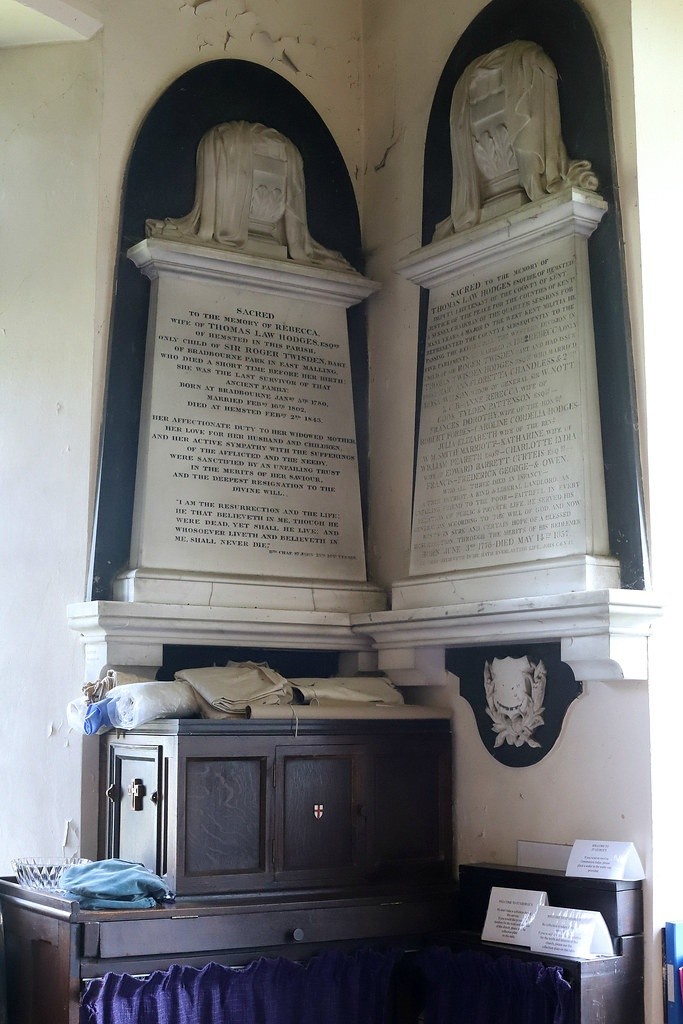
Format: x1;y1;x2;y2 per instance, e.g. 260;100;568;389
666;921;683;1024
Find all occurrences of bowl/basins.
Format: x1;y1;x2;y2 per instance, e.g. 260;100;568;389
11;856;93;898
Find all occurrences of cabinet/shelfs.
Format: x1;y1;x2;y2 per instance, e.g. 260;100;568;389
0;719;460;1024
